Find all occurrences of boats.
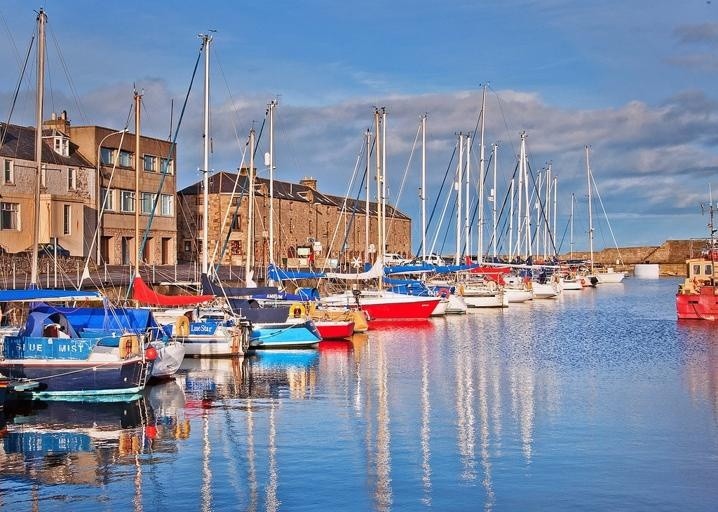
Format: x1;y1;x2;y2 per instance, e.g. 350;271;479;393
675;246;718;323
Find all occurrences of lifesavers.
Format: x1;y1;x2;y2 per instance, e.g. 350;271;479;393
119;333;138;358
290;302;305;318
458;286;464;296
176;316;189;336
581;278;586;284
437;288;449;299
